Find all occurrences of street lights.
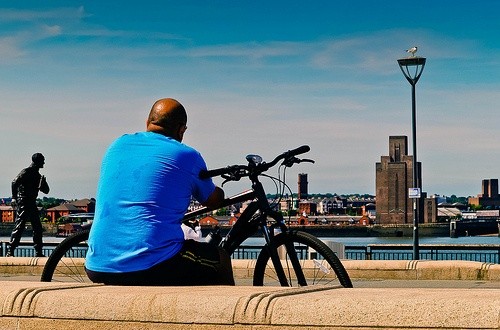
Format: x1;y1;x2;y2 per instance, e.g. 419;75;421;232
396;57;427;260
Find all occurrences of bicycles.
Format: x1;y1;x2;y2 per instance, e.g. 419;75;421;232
40;144;353;289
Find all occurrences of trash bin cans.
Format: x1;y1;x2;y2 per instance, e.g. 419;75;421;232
0;242;9;257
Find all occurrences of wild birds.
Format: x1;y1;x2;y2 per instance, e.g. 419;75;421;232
405;46;419;57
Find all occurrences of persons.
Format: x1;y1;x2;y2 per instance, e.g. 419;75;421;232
5;153;51;258
84;98;236;286
